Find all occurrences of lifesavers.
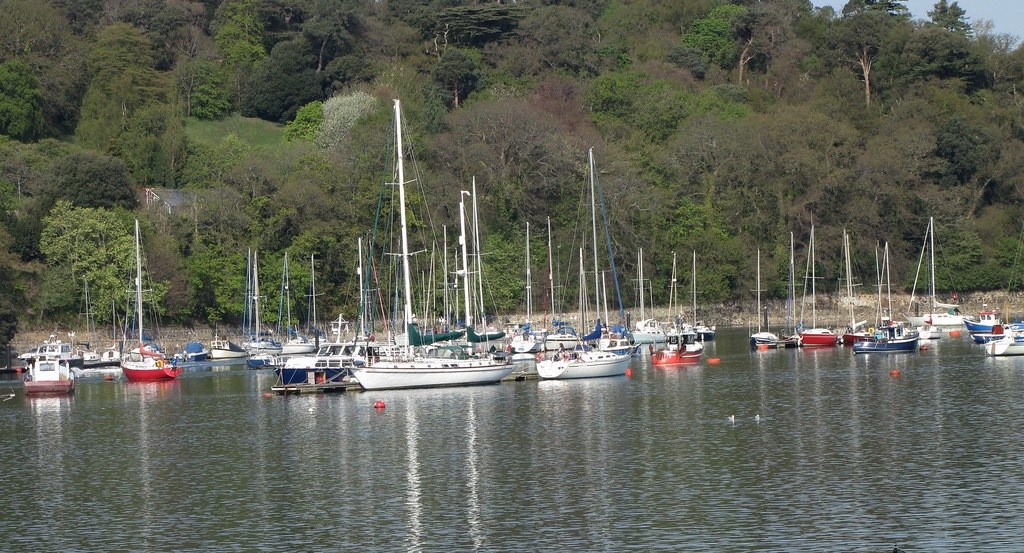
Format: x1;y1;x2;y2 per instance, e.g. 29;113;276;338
534;350;546;364
562;349;572;361
868;327;875;333
156;360;164;369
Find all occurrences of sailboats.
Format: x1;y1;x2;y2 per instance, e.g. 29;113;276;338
745;215;943;349
82;276;129;366
849;241;920;355
347;98;517;390
120;218;182;382
904;216;975;327
243;148;717;369
982;212;1024;357
272;238;396;385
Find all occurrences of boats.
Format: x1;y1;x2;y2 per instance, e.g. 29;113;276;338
172;342;208;362
21;355;75;396
16;332;84;369
207;322;247;359
963;302;1024;345
